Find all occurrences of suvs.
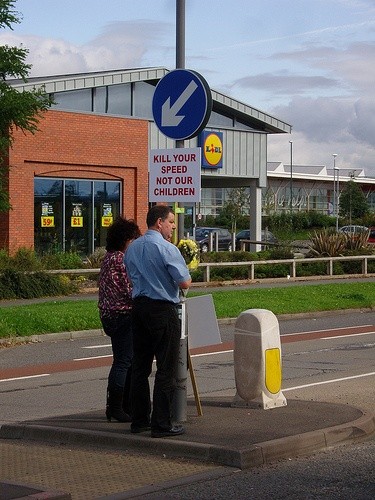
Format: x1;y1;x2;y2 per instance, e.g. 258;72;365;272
185;227;236;253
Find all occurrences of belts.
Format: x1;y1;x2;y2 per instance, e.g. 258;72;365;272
135;298;177;305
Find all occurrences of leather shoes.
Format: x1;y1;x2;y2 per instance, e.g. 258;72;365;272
151;424;185;438
131;425;151;433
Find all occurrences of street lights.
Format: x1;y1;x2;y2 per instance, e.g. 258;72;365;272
332;167;339;234
332;154;338;217
289;140;293;214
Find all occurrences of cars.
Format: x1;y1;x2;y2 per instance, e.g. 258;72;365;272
228;229;279;252
337;225;369;242
346;227;375;248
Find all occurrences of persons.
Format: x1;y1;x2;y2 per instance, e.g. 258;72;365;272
124;206;192;438
98;219;142;422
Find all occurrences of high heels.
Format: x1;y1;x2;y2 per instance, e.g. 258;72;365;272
106;413;132;423
